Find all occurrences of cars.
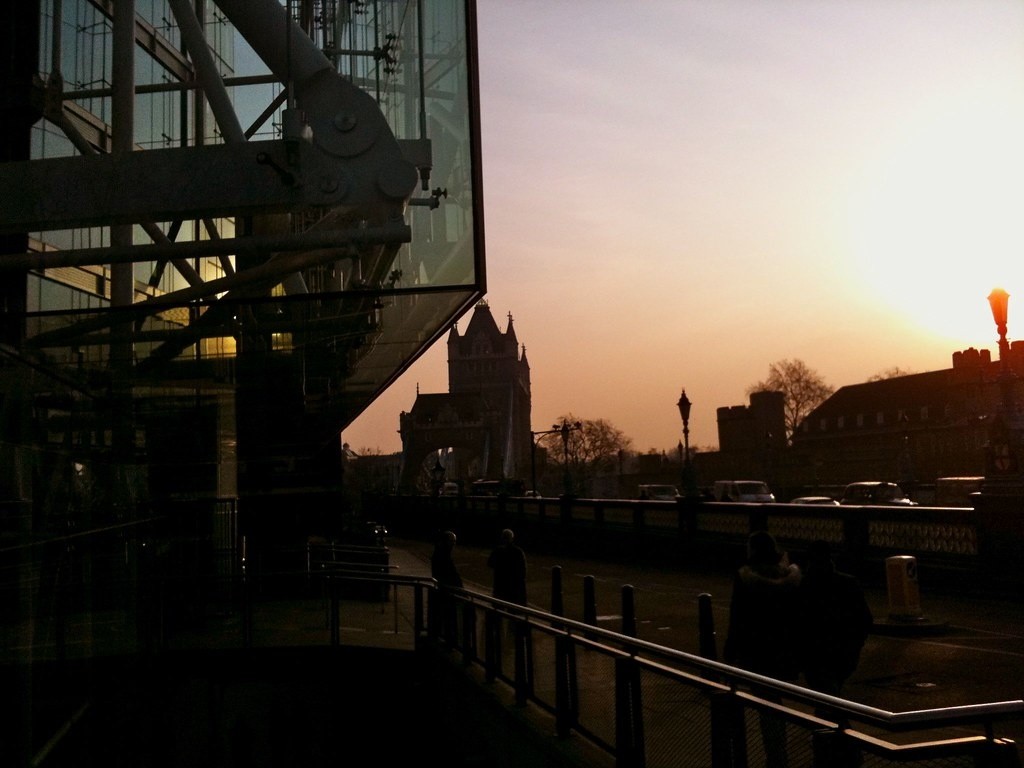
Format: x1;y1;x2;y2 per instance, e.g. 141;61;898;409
841;481;918;507
525;490;542;499
789;495;840;505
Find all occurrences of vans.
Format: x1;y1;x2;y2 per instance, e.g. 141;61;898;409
714;480;776;504
636;485;680;502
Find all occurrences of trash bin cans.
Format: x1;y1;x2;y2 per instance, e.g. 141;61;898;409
886;554;923;618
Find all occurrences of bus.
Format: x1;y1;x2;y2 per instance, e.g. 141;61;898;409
471;479;523;498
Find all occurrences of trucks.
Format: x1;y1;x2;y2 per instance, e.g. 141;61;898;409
439;483;458;494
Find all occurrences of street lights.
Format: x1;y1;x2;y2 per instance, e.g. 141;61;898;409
679;390;700;501
618;450;626;499
561;418;570;496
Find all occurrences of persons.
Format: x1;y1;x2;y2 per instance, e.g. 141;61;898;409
723;513;874;768
427;531;463;647
486;528;526;632
